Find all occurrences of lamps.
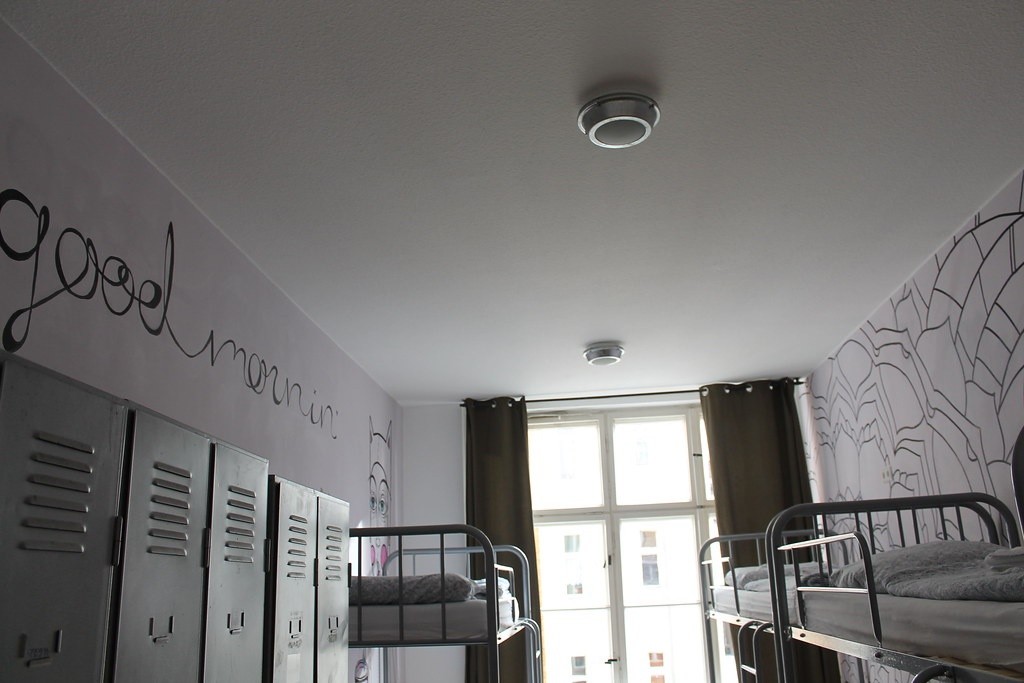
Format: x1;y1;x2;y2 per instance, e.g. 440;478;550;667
583;345;624;366
576;92;661;148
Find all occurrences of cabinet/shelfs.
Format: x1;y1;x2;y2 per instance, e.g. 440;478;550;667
0;353;351;683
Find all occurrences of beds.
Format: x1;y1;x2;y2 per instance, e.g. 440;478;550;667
696;492;1024;683
351;524;542;683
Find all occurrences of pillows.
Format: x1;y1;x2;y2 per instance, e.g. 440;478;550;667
350;568;474;605
724;559;832;591
